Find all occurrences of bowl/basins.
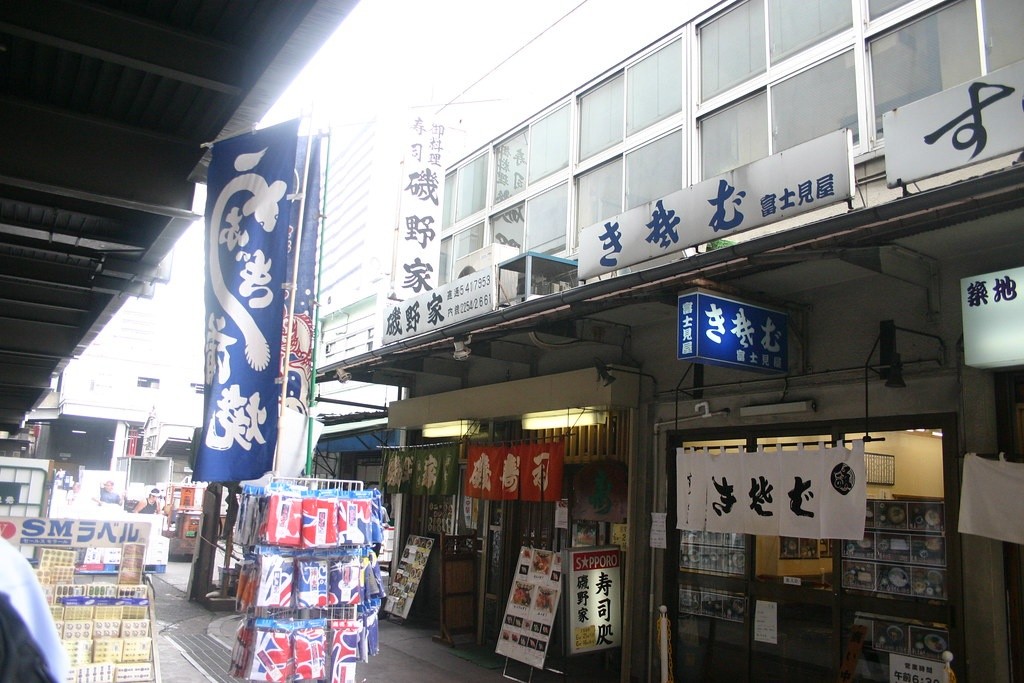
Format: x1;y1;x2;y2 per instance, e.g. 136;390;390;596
729;599;744;613
688;549;700;562
710;552;718;561
924;633;947;653
732;551;745;567
887;625;904;642
845;502;943;596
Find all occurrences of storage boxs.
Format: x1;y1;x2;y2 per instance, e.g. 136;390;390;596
34;542;154;683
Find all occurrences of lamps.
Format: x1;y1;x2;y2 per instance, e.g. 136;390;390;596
595;359;615;387
453;333;473;362
739;400;816;417
422;424;480;437
336;368;353;384
521;411;607;430
885;352;906;388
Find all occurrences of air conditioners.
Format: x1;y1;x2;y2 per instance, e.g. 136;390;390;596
454;244;519;309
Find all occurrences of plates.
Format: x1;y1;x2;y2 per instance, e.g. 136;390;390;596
416;569;422;578
428;502;453;533
410;569;416;578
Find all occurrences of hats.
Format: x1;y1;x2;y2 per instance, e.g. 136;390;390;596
150;488;160;496
104;481;114;486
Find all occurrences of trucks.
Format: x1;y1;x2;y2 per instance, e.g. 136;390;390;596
115;455;175;513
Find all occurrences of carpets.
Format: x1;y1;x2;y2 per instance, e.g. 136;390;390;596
448;646;522;668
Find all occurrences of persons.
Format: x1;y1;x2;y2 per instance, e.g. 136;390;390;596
100;481;126;506
132;489;161;514
56;467;81;505
374;506;390;557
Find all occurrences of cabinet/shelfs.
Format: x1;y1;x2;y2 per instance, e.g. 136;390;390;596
62;587;162;682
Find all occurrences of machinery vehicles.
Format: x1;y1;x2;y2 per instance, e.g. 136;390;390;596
160;484;224;560
